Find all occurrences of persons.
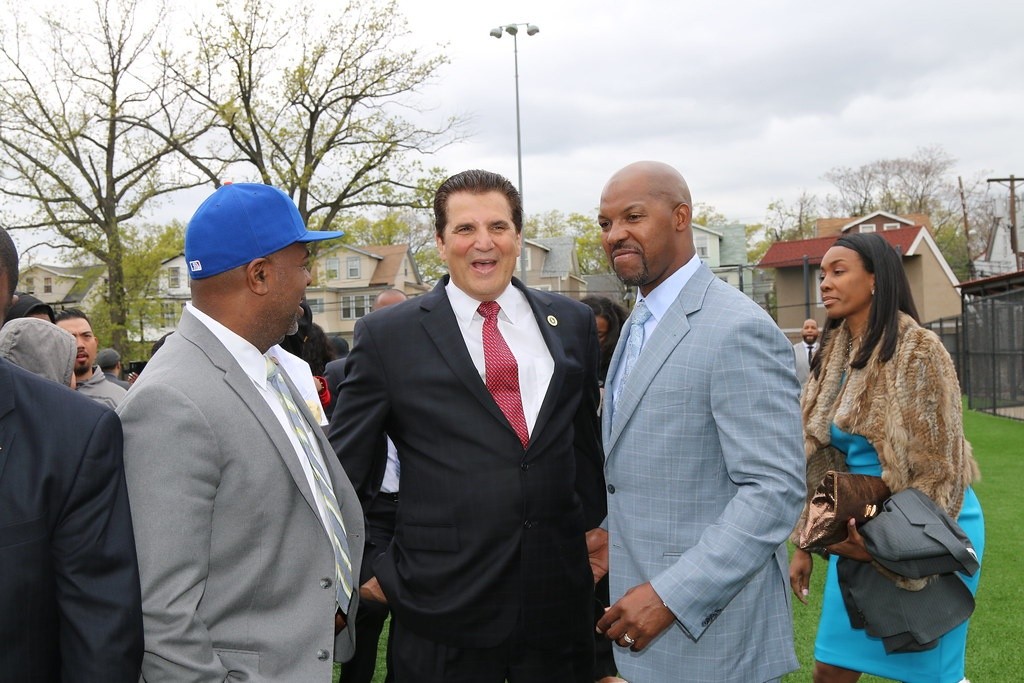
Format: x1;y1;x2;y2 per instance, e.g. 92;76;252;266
580;161;807;683
114;183;364;683
0;226;825;683
324;171;622;683
788;233;985;683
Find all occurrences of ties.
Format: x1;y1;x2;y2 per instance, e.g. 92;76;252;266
807;345;814;366
475;301;530;451
611;302;652;436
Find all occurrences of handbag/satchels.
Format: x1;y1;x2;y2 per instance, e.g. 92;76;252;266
800;470;893;554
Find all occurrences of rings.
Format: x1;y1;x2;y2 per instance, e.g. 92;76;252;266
623;634;636;644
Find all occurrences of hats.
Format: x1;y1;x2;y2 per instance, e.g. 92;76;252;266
186;183;343;280
97;348;121;367
